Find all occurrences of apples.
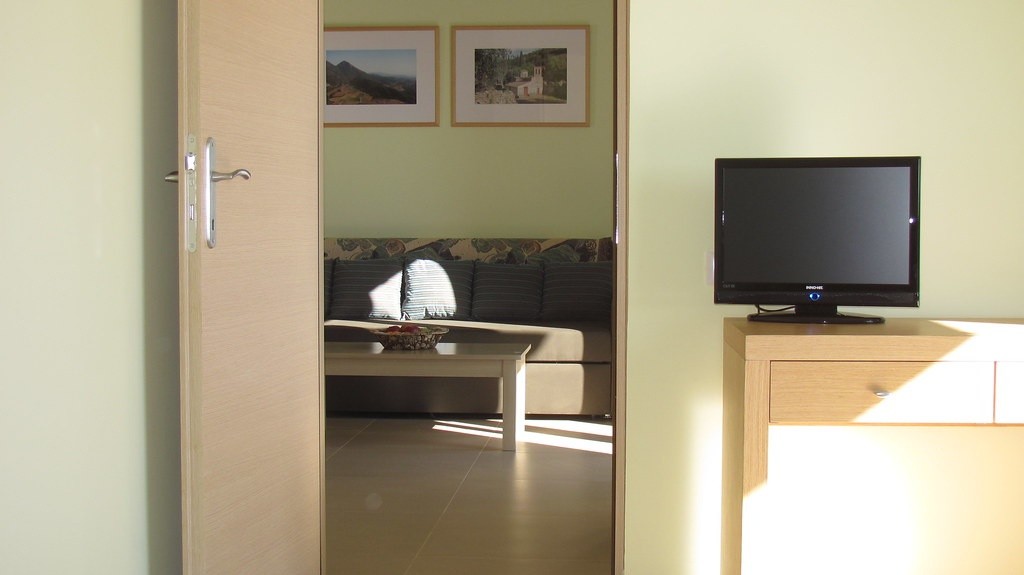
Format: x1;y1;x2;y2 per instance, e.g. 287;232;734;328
384;324;420;333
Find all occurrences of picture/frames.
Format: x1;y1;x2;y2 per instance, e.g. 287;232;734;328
322;26;440;127
450;24;590;128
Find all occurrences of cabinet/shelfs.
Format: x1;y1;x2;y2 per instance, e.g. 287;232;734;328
721;317;1024;575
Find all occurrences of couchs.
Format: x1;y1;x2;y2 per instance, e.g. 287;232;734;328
323;236;614;421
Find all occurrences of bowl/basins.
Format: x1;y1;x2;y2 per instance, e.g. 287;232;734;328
370;328;449;350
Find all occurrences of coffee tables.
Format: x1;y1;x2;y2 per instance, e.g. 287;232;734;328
324;341;531;452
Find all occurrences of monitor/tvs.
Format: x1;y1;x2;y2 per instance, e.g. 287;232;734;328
716;157;922;326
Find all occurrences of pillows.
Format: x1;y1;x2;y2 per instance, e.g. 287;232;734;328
543;261;612;325
324;257;335;322
472;258;544;325
331;259;404;320
401;256;474;322
